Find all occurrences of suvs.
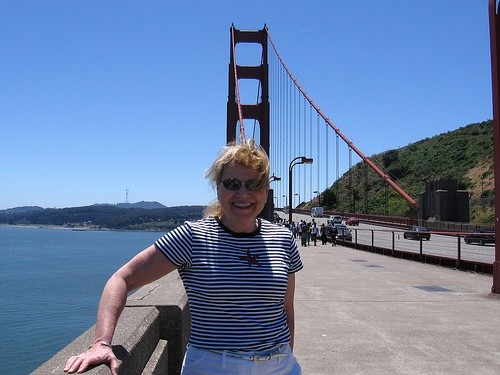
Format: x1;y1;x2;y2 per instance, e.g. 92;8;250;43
328;214;342;223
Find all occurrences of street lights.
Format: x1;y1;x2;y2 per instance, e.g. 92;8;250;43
268;175;281;188
313;191;320;207
289;156;313;224
274;197;278;208
295;193;300;214
283;196;287;206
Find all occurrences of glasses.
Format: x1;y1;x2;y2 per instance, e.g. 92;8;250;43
220;177;266;192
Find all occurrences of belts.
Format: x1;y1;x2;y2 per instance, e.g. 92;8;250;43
209;344;287;361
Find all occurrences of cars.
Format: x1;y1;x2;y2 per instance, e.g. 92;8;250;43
464;229;495;246
403;226;431;242
346;217;360;227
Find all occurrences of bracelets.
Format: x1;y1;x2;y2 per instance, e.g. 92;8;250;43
88;342;112;349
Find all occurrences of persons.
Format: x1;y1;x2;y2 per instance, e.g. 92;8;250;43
272;213;338;248
64;140;304;375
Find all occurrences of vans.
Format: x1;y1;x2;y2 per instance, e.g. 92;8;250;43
329;223;351;241
281;205;294;215
309;207;324;218
